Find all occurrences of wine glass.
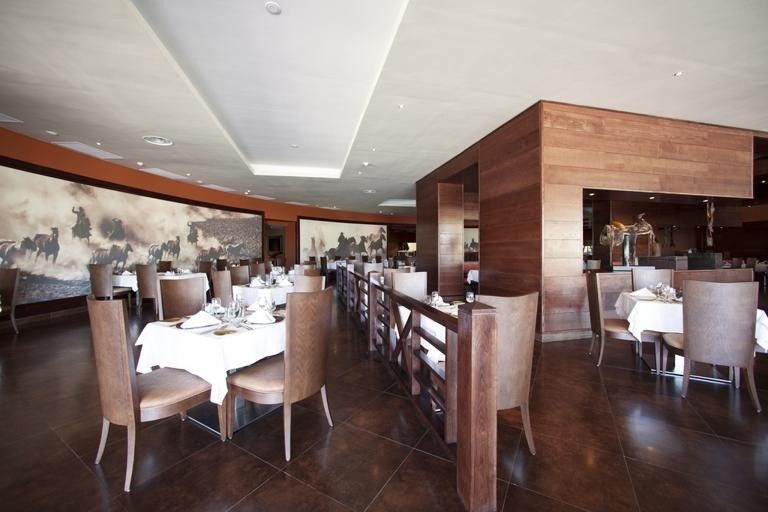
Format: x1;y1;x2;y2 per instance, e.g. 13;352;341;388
651;282;680;303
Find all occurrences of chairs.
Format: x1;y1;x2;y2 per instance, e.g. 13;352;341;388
0;266;21;336
228;283;337;465
82;291;228;494
87;253;391;321
680;278;762;415
370;265;429;364
582;268;662;376
428;290;540;458
581;248;767;296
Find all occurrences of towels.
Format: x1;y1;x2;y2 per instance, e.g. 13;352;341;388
179;310;222;330
629;287;656;299
244;306;276;324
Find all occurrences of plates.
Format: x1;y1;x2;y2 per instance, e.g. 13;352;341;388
172;291;284;336
243;282;294;289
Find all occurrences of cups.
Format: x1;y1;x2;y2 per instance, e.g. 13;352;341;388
464;292;475;303
410;262;414;267
430;290;440;304
